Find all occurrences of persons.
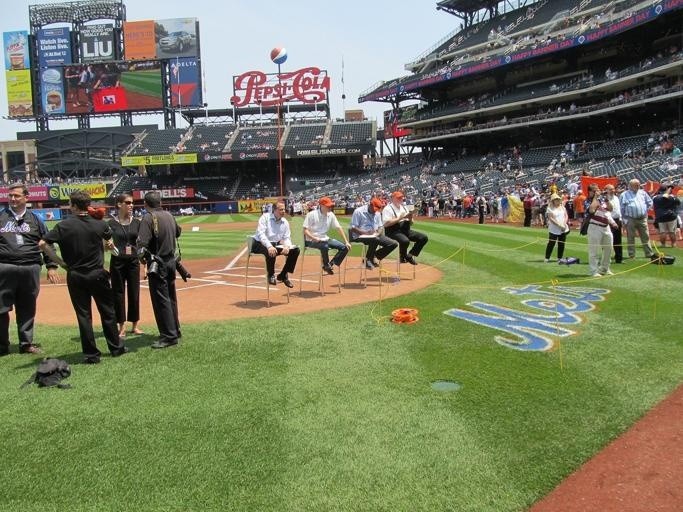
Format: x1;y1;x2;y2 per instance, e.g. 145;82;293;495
37;191;130;363
2;25;682;278
102;192;142;336
249;200;300;290
0;181;64;357
134;188;182;349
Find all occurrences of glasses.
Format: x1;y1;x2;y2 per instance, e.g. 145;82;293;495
125;201;137;205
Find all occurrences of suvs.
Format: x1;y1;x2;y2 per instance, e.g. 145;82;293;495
158;31;191;53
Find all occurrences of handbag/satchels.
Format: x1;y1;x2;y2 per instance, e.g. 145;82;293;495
580;219;588;235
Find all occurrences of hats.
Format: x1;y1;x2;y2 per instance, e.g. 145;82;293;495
370;191;404;212
319;196;334;207
550;192;561;201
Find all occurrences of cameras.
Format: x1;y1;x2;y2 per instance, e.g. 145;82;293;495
175;252;191;282
598;189;607;195
146;254;161;276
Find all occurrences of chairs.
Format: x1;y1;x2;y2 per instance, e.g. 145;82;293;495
110;2;681;216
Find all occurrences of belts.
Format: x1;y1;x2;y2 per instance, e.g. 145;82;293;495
588;222;609;227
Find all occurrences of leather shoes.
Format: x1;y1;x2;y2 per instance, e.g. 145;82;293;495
323;263;333;274
268;273;294;288
151;336;179;348
400;256;418;265
23;344;45;354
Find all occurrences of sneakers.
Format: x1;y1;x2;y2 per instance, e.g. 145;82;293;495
365;258;379;269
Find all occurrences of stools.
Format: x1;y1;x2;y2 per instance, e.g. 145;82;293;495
244;234;289;307
299;233;341;297
344;228;381;288
382;236;416;281
